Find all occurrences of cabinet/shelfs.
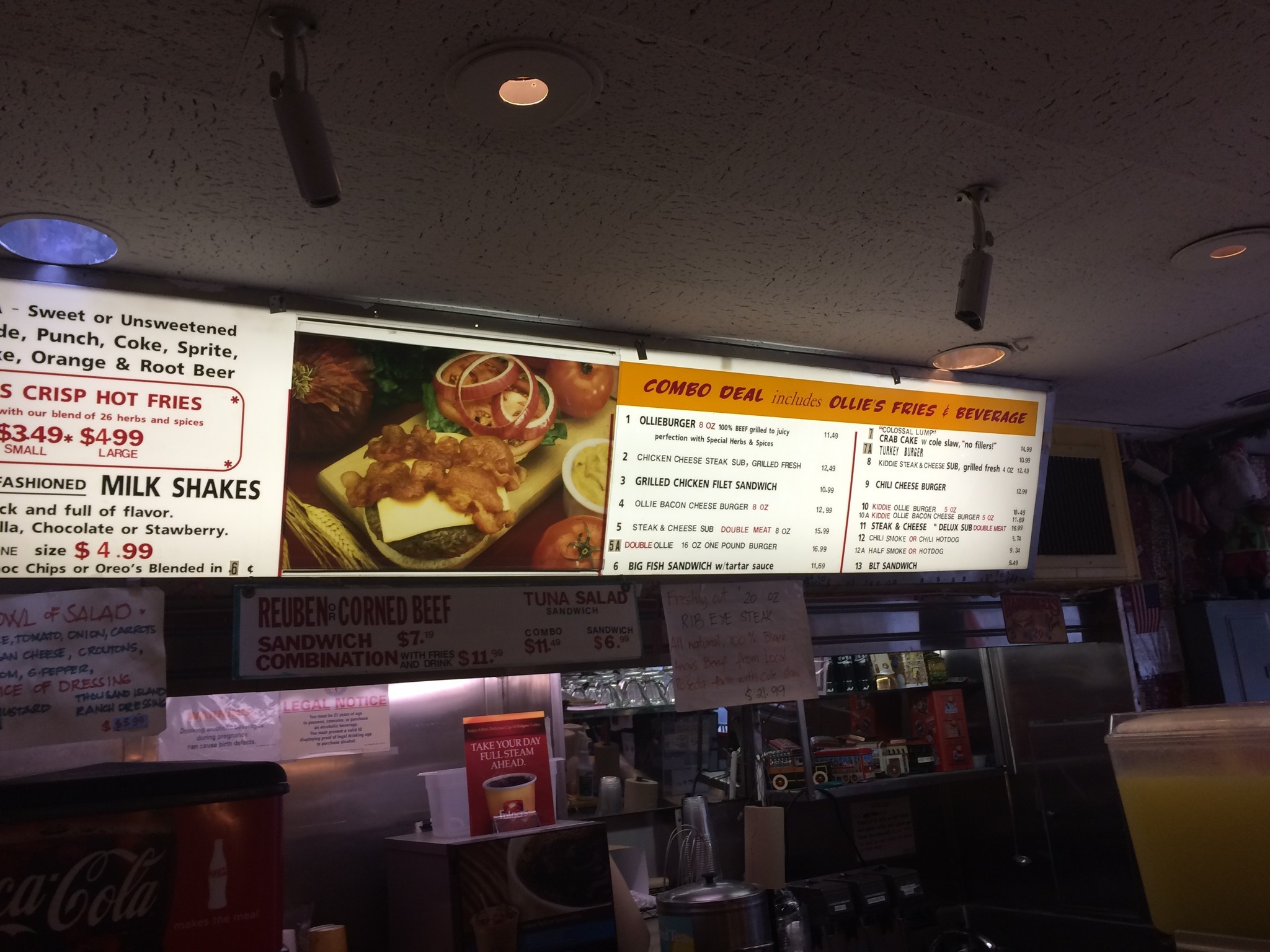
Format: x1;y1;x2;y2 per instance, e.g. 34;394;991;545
746;646;1006;804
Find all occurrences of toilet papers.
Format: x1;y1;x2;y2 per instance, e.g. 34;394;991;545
744;805;786;891
623;778;659;816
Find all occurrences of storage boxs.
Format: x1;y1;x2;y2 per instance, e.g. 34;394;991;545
868;645;974;776
616;716;723;796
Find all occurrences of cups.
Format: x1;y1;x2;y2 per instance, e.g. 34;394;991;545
682;795;716;882
561;438;610;520
482;773;537;820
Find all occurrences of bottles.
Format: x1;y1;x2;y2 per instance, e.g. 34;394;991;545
207;839;227;909
595;776;623;817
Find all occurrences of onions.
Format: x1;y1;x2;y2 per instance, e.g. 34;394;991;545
289;340;375;454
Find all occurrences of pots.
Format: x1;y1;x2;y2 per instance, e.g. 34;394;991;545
656;872;775;952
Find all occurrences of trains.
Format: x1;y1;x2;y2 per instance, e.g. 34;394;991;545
807;748;876;783
848;739;912;777
759;749;828;791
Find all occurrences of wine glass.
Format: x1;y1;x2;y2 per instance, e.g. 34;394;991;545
557;666;675;708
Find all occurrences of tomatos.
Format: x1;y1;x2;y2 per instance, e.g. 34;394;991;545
436;351;615;458
530;515;604;571
